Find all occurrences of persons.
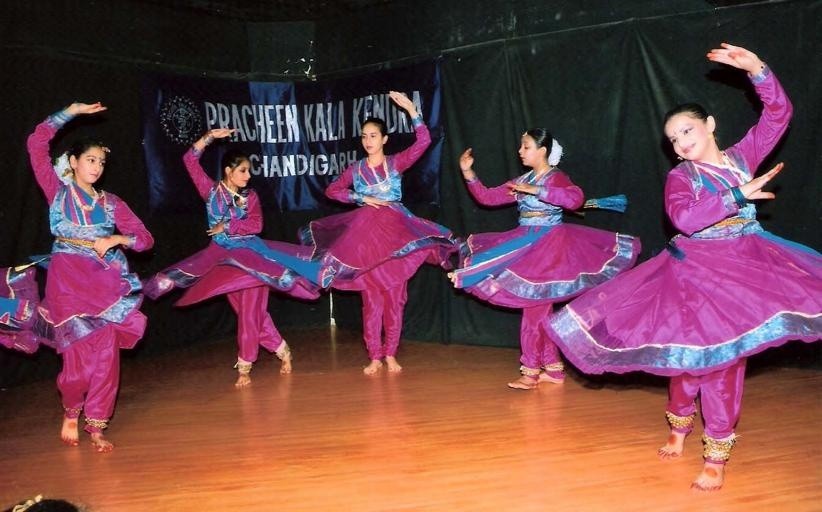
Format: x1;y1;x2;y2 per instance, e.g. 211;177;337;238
540;42;822;491
446;128;641;390
143;128;324;386
298;91;463;376
1;101;157;452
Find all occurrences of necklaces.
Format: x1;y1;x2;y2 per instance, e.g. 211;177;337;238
69;183;100;210
690;157;753;182
220;181;247;207
509;170;543;195
365;157;391;193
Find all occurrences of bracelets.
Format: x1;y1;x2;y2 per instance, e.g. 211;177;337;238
731;186;746;208
203;130;216;146
55;107;75;121
